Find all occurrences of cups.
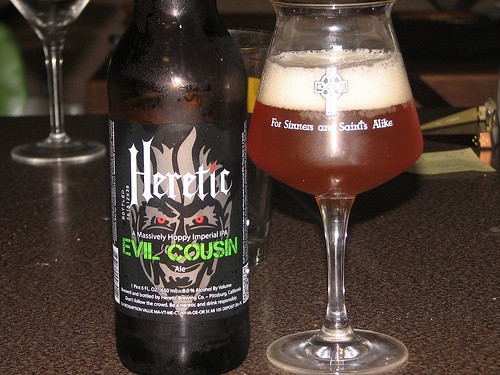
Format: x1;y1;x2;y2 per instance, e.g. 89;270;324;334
224;30;274;266
10;0;104;164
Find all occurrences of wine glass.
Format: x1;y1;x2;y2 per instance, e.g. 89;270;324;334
246;0;423;375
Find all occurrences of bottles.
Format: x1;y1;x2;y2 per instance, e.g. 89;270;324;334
106;0;252;374
